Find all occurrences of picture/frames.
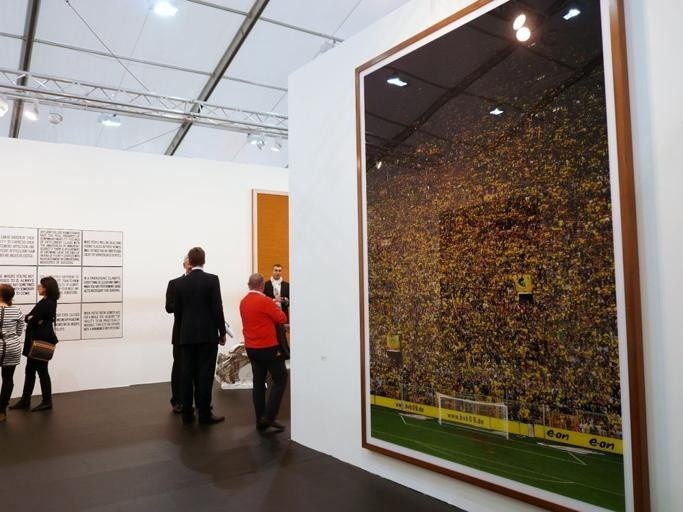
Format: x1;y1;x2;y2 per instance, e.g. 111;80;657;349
252;188;289;283
354;0;651;512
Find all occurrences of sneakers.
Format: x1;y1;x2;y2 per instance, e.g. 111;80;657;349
0;413;8;421
256;417;285;431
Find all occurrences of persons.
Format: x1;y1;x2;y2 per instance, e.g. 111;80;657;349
240;273;288;433
0;283;25;422
172;247;226;424
8;276;60;411
263;264;289;337
166;255;213;414
367;84;623;440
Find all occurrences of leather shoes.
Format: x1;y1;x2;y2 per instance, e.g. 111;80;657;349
8;400;31;409
172;402;224;425
31;400;53;412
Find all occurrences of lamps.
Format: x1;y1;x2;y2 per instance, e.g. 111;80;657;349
246;127;282;152
0;92;121;127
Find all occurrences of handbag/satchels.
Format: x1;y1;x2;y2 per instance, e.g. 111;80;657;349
28;339;56;361
